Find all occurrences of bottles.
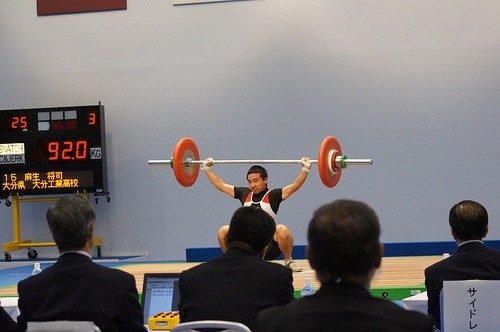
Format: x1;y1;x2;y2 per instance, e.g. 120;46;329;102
301;278;315;297
32;263;42;276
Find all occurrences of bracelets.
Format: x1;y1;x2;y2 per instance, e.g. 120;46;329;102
302;167;310;172
204;167;210;171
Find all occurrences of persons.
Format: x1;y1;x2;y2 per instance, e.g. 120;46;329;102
256;200;433;332
0;301;19;332
203;157;312;271
17;195;148;332
424;200;500;329
177;206;294;332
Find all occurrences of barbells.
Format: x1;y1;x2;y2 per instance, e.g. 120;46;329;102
148;138;374;189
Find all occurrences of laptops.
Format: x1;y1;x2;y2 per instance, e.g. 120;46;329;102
141;272;183;325
